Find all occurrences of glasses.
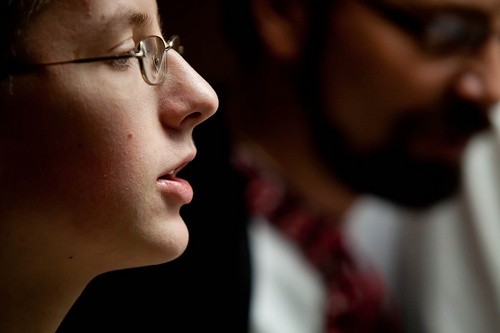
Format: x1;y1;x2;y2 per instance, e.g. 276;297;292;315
359;0;500;60
5;35;184;86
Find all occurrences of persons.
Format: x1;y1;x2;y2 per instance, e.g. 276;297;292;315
1;1;220;333
156;0;499;333
